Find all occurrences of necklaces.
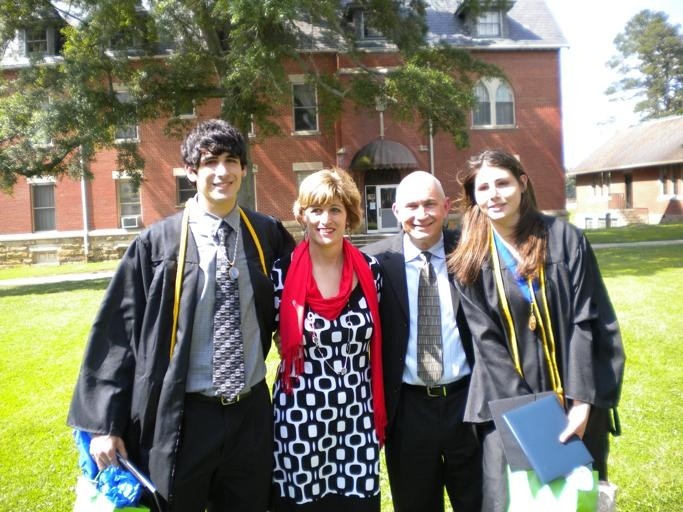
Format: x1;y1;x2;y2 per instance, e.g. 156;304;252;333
525;278;539;332
206;223;242;283
303;302;351;376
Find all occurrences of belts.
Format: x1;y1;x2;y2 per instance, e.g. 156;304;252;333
424;386;451;398
218;395;240;406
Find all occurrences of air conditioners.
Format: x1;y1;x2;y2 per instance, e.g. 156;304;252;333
120;215;142;229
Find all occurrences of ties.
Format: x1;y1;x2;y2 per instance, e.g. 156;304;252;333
418;251;444;387
213;225;246;403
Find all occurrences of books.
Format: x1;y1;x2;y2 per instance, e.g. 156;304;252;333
500;395;595;485
111;452;163;511
486;389;565;473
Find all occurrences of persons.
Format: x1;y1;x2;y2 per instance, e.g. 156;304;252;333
442;146;627;512
63;115;299;511
355;168;487;511
259;164;388;510
